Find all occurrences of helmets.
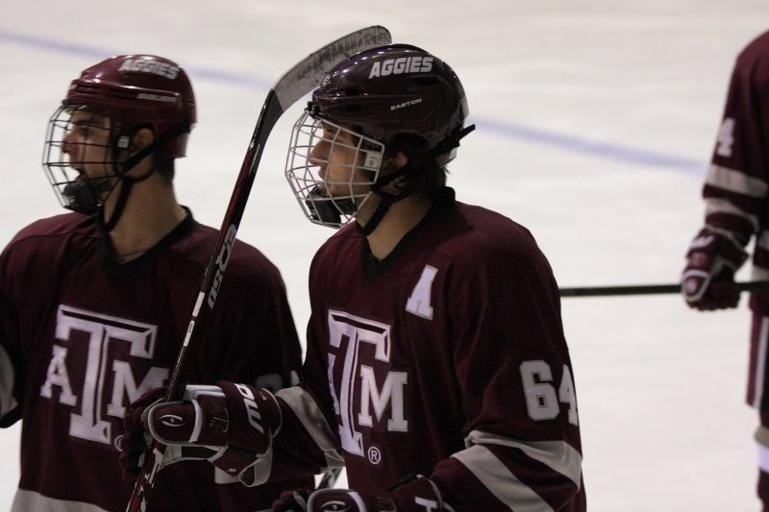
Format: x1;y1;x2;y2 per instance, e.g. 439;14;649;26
304;44;469;167
68;53;197;158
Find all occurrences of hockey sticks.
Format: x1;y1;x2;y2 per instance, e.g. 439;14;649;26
126;25;392;511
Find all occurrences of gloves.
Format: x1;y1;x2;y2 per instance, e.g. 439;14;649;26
680;248;742;312
272;475;443;512
117;380;282;487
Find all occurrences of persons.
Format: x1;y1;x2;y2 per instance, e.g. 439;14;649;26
682;30;769;512
